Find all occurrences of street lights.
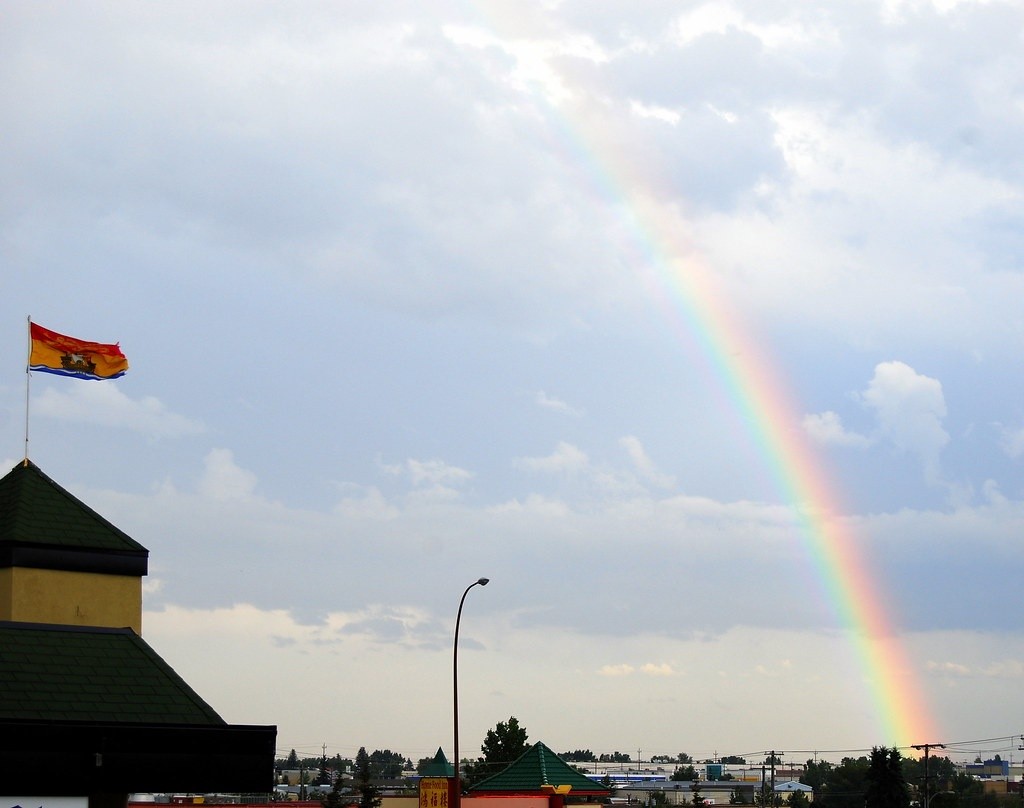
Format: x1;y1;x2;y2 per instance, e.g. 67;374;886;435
451;577;490;808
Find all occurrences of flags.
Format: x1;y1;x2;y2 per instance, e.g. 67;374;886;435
27;321;131;383
